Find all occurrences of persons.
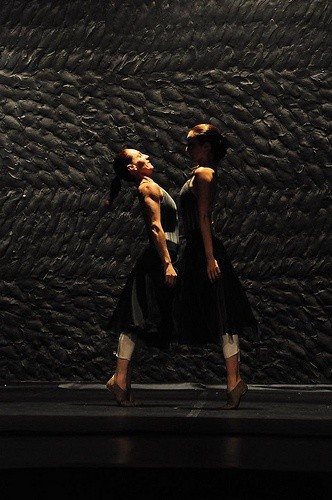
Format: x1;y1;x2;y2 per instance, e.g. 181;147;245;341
105;148;178;407
175;123;253;409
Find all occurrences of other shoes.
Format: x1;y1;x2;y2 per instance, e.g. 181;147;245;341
105;374;136;407
224;380;248;409
